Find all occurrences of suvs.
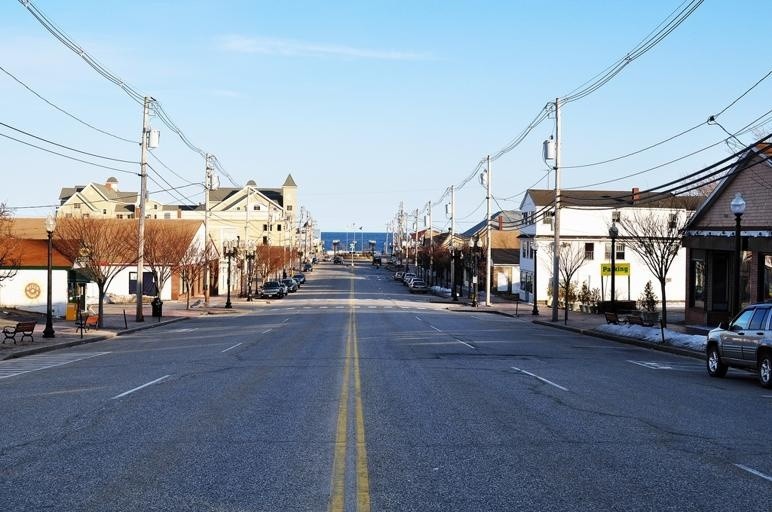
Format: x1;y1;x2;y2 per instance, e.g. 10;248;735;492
705;304;772;389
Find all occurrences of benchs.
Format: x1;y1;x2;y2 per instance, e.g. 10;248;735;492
604;311;654;327
2;320;37;345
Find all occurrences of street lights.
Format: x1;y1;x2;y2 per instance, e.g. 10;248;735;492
245;242;257;301
531;242;539;315
608;223;618;311
224;240;237;309
468;235;483;309
729;192;746;318
448;239;463;301
44;216;59;337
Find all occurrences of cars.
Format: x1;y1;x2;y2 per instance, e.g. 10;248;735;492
393;272;428;293
261;273;305;298
304;263;313;271
334;256;344;264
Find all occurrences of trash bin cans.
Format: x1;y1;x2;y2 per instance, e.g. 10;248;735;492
67;303;78;320
151;298;162;317
